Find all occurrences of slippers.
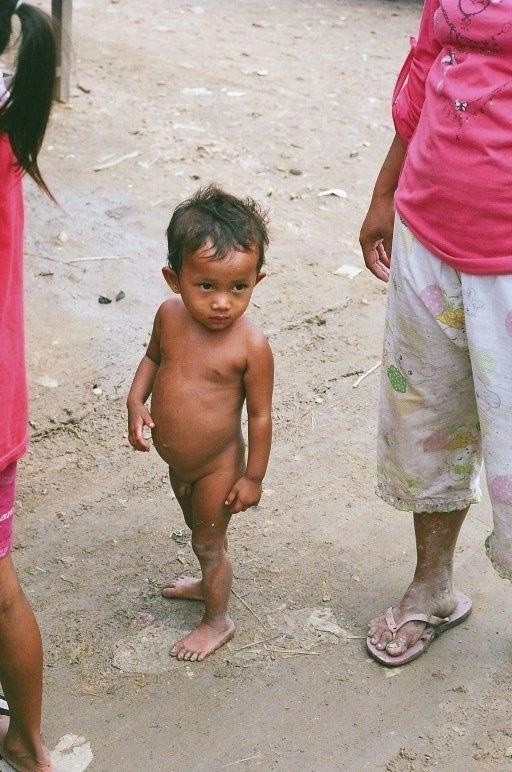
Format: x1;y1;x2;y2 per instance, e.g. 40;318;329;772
368;590;472;668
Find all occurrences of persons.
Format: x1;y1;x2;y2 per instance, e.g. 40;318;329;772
125;181;279;664
1;2;60;772
358;1;512;666
